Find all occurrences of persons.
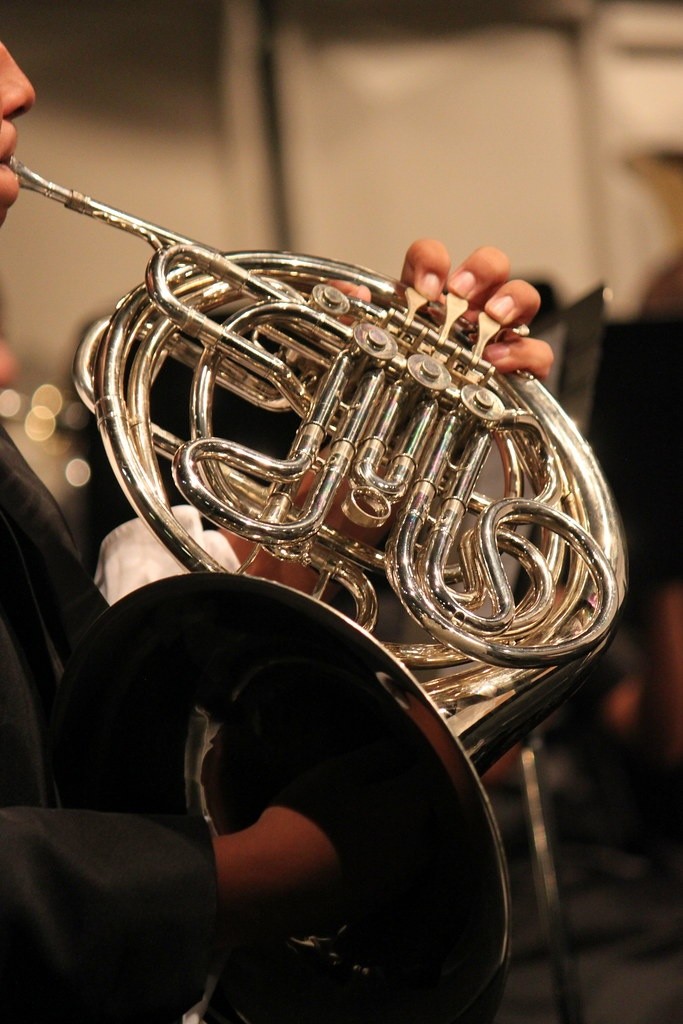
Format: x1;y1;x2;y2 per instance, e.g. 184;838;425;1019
1;34;682;1023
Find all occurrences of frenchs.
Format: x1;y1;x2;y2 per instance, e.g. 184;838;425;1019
10;150;638;1024
